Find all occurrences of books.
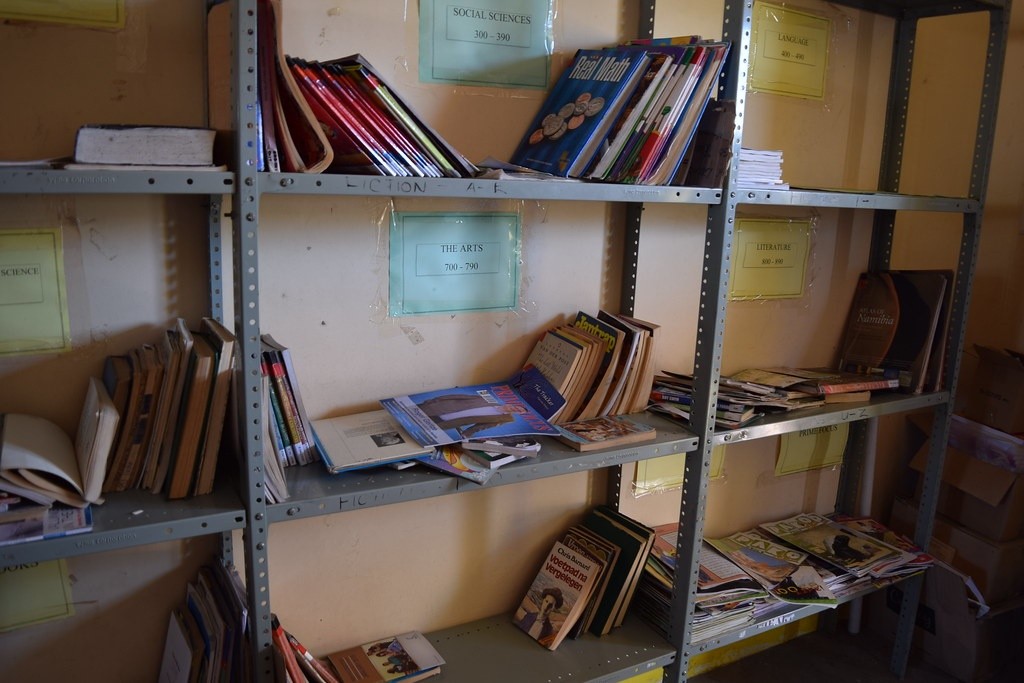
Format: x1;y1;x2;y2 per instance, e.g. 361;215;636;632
0;272;658;683
646;510;933;646
66;122;231;173
737;148;792;191
650;267;955;432
257;0;475;178
509;34;732;185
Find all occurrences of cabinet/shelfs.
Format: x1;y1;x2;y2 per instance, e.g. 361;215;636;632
0;1;1015;682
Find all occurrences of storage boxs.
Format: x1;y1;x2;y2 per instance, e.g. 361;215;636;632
862;342;1024;683
